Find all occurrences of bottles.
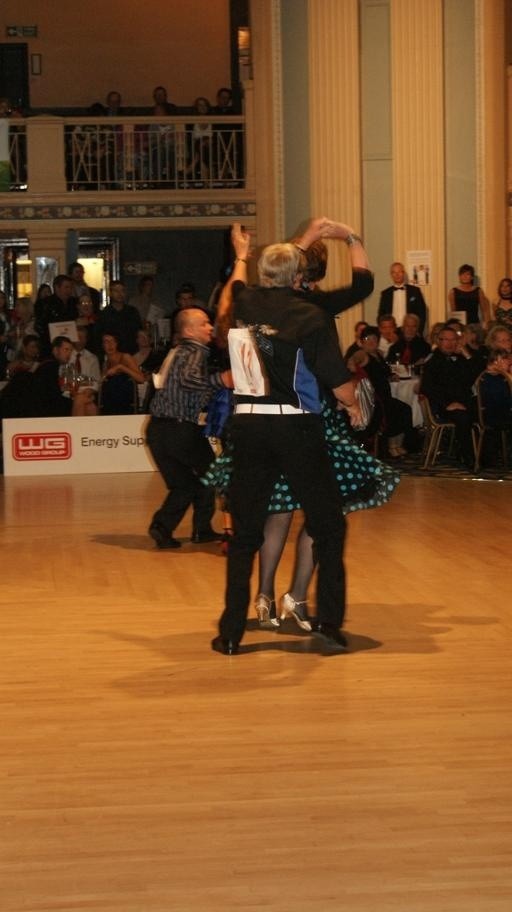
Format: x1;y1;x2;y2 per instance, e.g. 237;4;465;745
62;363;79;400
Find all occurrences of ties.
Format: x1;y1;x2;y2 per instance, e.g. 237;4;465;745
74;352;82;376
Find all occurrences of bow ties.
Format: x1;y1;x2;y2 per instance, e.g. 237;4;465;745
393;285;404;291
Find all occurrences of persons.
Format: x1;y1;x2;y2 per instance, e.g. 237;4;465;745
144;307;237;550
252;214;376;634
343;262;512;474
211;219;253;557
254;219;364;657
1;257;205;419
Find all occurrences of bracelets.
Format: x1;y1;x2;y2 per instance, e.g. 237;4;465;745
342;233;365;248
293;241;308;255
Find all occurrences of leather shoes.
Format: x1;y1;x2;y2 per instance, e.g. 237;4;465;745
388;445;407;459
211;635;240;656
312;619;349;648
148;521;181;549
192;531;226;543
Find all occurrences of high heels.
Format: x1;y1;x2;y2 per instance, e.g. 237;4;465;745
254;592;280;629
280;590;312;633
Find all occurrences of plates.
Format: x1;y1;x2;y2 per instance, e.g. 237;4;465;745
400;376;412;380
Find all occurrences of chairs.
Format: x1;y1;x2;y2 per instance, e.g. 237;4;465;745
97;372;138;415
419;367;512;475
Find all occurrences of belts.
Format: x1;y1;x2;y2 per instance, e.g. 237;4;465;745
232;403;312;414
